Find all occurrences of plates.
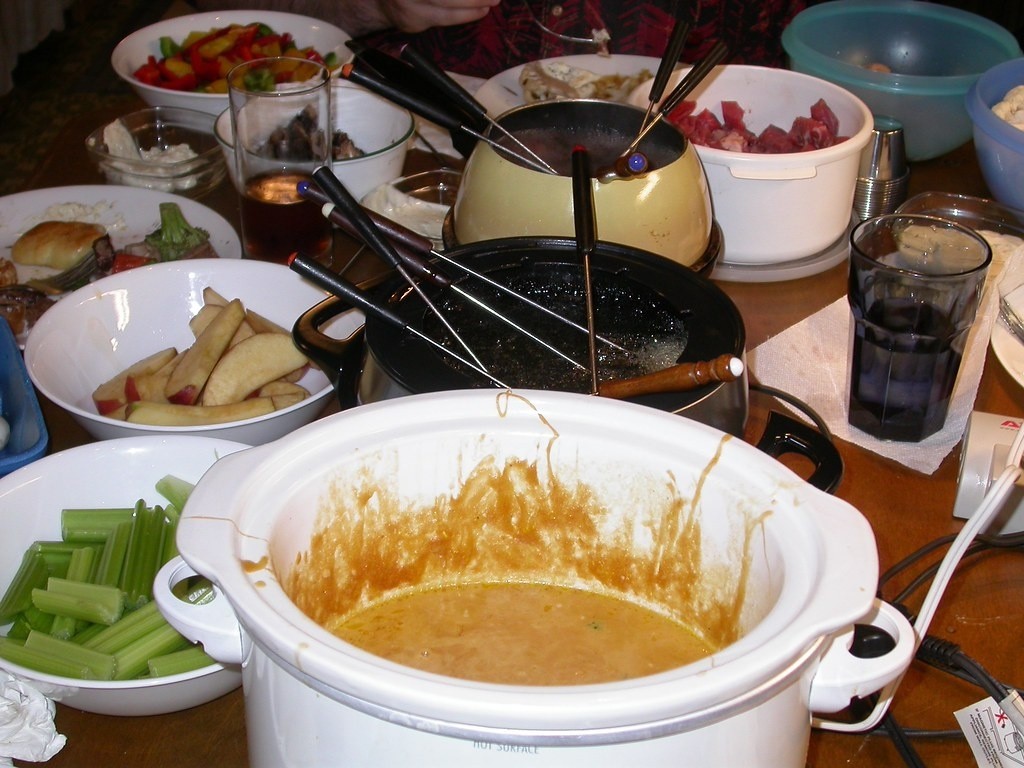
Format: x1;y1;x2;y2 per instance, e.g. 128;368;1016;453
696;206;860;283
989;281;1024;389
465;54;695;119
0;185;242;350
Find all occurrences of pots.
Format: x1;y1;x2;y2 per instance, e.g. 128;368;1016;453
441;98;724;276
152;389;915;768
291;235;843;492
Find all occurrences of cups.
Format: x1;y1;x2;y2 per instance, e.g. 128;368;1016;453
847;213;993;443
853;115;910;221
226;55;335;272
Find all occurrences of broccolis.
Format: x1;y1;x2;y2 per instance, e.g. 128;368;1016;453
143;202;210;261
519;63;574;102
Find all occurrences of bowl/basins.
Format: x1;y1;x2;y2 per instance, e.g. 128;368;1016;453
964;57;1024;211
110;10;356;134
212;77;415;209
779;0;1021;161
85;105;228;200
624;63;874;266
0;435;256;716
24;258;366;445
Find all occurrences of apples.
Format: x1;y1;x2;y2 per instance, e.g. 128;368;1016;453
91;286;320;427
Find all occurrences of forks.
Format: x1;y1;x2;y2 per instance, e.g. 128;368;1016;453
999;294;1024;344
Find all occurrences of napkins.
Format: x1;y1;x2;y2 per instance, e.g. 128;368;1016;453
746;283;1000;476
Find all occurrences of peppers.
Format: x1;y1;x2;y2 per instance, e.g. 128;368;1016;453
133;21;337;95
